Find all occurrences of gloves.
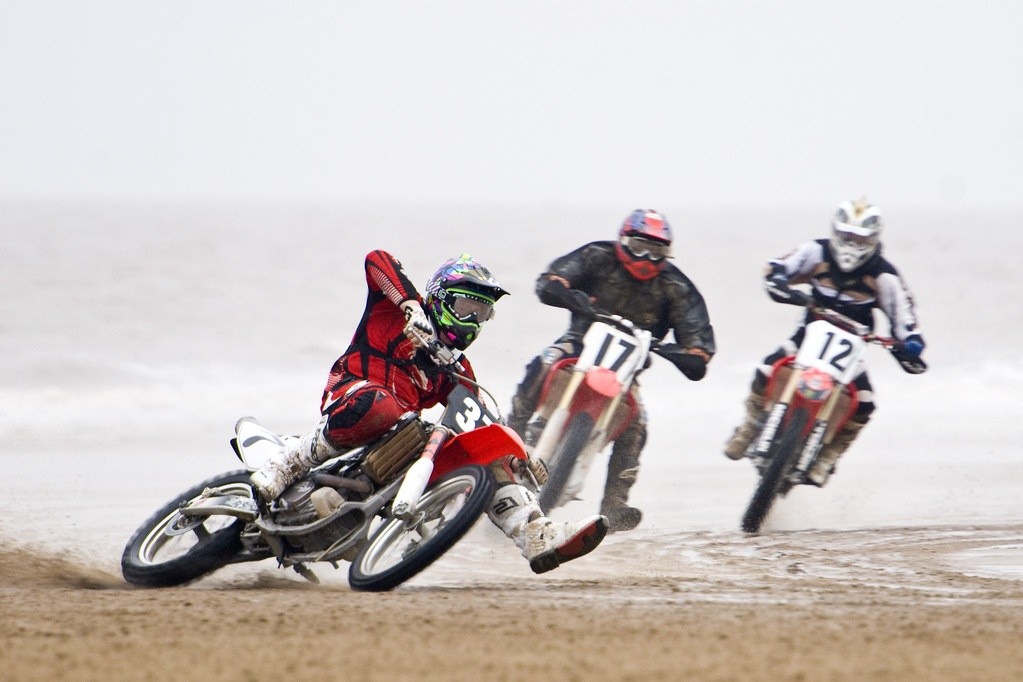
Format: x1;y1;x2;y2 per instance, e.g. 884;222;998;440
402;305;435;347
903;338;923;356
508;454;549;487
768;267;789;292
551;275;570;289
688;347;712;364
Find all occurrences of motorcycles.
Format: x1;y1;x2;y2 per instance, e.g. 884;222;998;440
120;320;550;594
741;274;928;535
526;280;706;522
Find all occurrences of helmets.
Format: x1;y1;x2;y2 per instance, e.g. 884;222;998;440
831;198;885;270
425;254;511;351
615;208;674;280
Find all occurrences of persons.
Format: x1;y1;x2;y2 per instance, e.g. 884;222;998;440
509;209;715;533
249;250;609;574
724;197;925;486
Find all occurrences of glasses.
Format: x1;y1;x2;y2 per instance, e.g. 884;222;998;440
831;228;877;256
621;235;667;262
441;289;495;330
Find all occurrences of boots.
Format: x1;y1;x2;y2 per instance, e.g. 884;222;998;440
249;413;343;501
505;393;538;440
807;417;866;488
723;392;771;460
485;484;609;574
599;451;641;533
